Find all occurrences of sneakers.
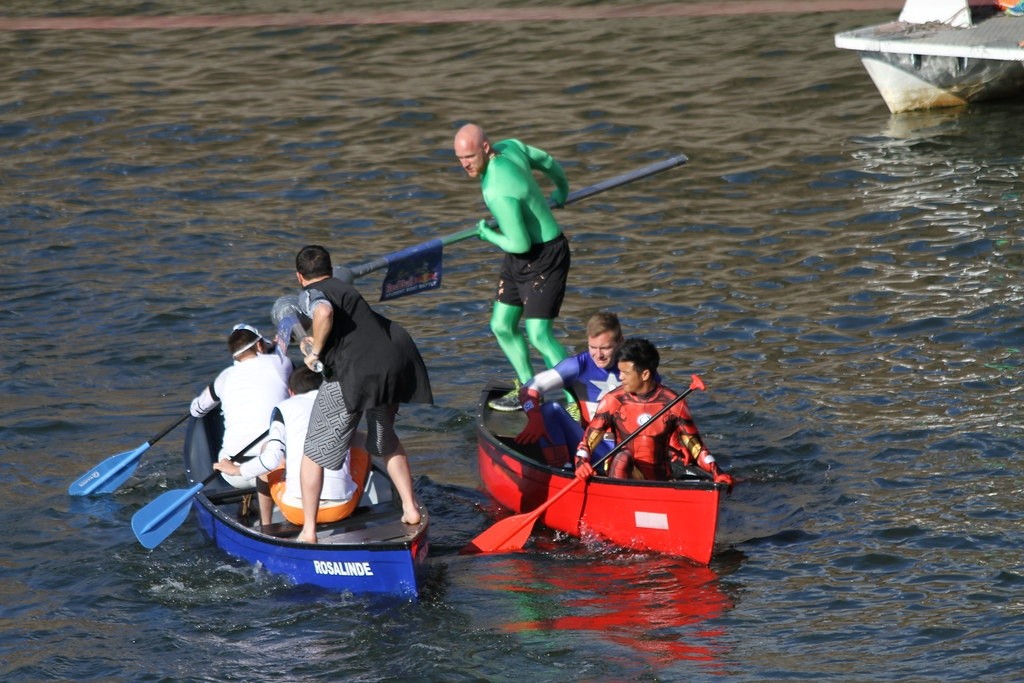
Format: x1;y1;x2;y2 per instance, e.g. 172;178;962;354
565;401;581;423
488;379;526;411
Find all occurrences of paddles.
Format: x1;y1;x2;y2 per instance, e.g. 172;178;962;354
459;373;706;554
132;428;269;550
68;335;296;495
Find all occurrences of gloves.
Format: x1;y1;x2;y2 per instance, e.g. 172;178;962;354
516;418;553;446
575;460;598;480
713;471;733;485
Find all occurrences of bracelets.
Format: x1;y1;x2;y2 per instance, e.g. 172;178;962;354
311;352;320;357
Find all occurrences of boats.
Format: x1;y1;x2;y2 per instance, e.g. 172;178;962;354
182;407;429;601
477;374;726;568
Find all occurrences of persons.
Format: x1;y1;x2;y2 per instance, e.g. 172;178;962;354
295;245;435;542
189;330;294;488
575;338;735;494
514;312;662;468
454;124;581;422
213;367;371;525
